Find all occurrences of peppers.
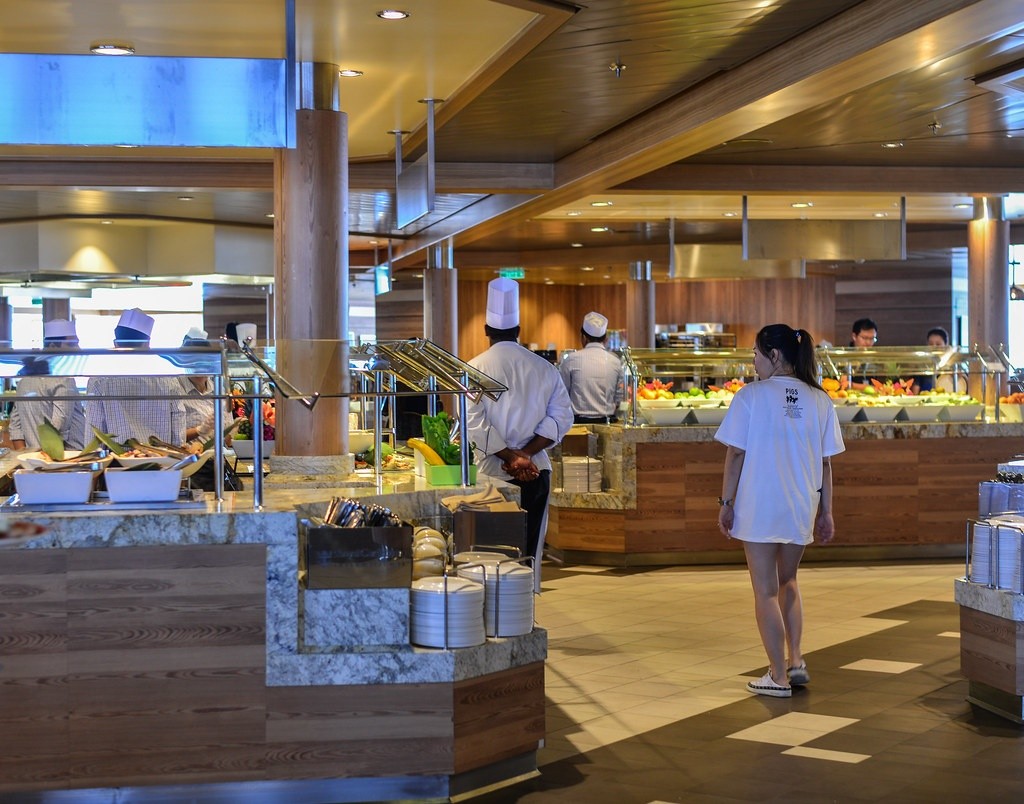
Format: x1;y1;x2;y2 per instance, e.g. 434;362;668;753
445;441;477;465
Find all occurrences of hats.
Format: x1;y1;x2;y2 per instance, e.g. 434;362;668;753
117;307;155;337
186;327;208;340
237;323;257;348
583;312;608;337
486;277;519;329
44;319;76;337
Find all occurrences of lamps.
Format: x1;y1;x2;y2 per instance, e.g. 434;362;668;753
1010;263;1024;300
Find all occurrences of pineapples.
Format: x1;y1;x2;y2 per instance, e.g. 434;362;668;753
236;375;261;439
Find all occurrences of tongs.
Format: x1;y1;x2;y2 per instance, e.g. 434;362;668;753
127;435;200;470
54;450;110;462
34;462;103;473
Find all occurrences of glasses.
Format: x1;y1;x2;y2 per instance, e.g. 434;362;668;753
858;335;877;342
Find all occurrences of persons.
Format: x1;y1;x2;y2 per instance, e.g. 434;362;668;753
559;311;624;423
833;318;899;386
8;308;237;450
459;301;574;597
909;327;949;394
382;337;444;441
179;321;258;354
714;324;847;698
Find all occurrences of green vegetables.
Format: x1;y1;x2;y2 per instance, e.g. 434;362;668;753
420;412;453;458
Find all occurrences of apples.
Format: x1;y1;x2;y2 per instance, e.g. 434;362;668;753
863;386;875;395
636;388;717;400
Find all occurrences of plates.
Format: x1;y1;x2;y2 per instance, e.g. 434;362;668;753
562;457;602;493
410;552;535;649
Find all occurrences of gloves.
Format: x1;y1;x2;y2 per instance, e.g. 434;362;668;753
195;415;215;436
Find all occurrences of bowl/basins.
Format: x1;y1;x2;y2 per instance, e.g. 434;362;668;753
111;448;215;477
16;451;114;472
411;527;447;579
349;432;375;453
637;393;986;426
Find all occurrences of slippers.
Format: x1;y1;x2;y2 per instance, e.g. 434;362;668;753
786;659;810;685
747;664;792;697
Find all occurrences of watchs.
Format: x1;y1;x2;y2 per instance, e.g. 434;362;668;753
717;496;734;505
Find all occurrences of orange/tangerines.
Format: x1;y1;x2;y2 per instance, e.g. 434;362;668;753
999;391;1024;404
821;378;847;399
